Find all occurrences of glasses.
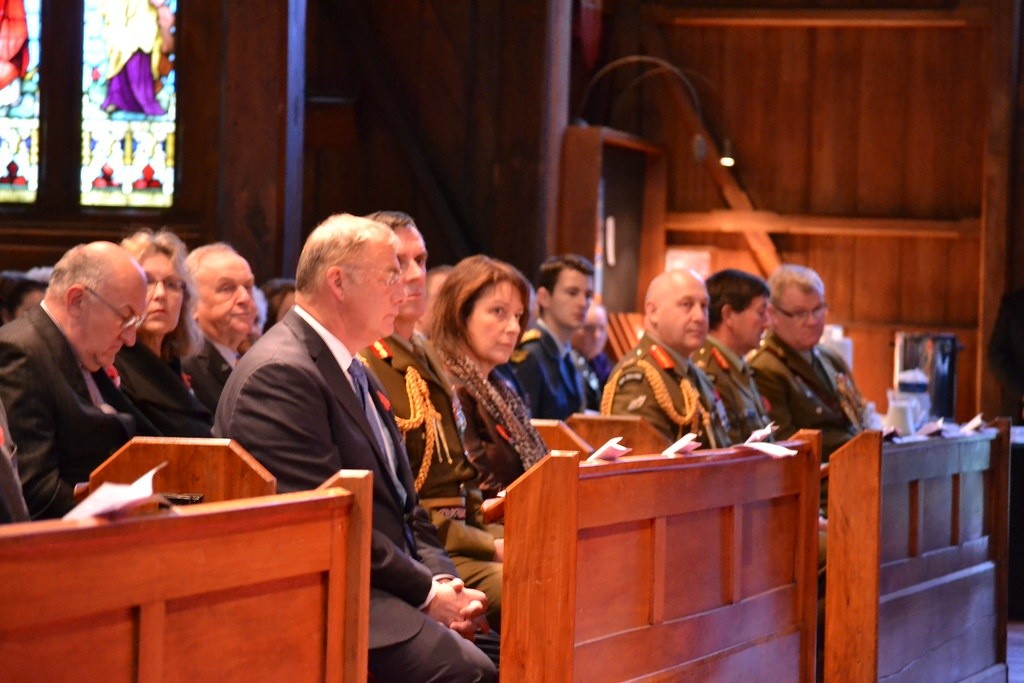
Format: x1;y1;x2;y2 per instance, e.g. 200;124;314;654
136;272;187;291
369;270;402;289
84;285;146;331
771;302;828;320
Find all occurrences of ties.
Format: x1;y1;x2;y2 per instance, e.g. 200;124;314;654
347;356;395;485
411;343;429;370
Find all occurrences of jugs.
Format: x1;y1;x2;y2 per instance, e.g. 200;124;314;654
882;391;922;435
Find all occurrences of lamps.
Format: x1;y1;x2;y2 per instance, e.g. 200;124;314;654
719;138;735;167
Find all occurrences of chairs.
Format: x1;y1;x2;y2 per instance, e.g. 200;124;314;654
0;414;1024;683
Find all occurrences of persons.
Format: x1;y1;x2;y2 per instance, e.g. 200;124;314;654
506;254;614;421
605;269;734;450
746;264;884;520
427;253;548;502
691;269;829;566
208;213;499;683
357;211;505;621
0;227;299;524
